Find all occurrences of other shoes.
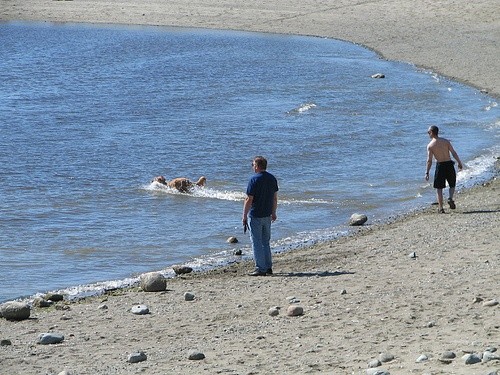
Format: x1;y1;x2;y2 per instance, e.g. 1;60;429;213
437;208;445;214
248;267;273;276
447;197;457;210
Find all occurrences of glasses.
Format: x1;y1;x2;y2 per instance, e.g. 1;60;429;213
427;130;432;133
251;163;257;166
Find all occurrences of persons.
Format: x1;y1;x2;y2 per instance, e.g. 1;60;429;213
242;156;278;276
425;126;462;214
